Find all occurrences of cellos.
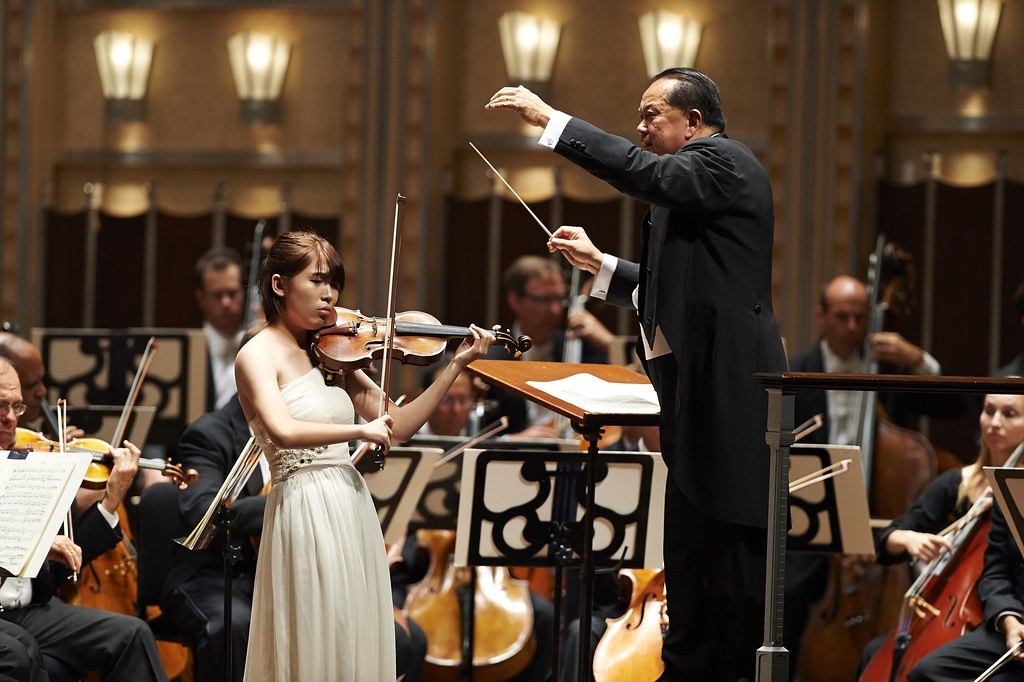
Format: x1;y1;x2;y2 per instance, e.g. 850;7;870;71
853;439;1024;682
790;234;965;682
41;395;196;682
503;248;627;606
400;524;540;682
587;564;670;682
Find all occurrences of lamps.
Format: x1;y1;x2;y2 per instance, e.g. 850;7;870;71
225;28;292;119
935;0;1003;81
91;28;155;120
636;8;705;83
497;11;562;106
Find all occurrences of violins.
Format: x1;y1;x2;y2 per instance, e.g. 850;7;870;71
313;306;530;372
0;427;199;492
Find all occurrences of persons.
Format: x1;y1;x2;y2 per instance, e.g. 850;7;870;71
784;275;1024;682
0;231;662;682
485;66;793;682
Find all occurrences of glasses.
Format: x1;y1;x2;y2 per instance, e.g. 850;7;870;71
522;292;571;309
0;403;27;416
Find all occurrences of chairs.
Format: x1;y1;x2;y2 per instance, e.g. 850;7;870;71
131;483;200;682
